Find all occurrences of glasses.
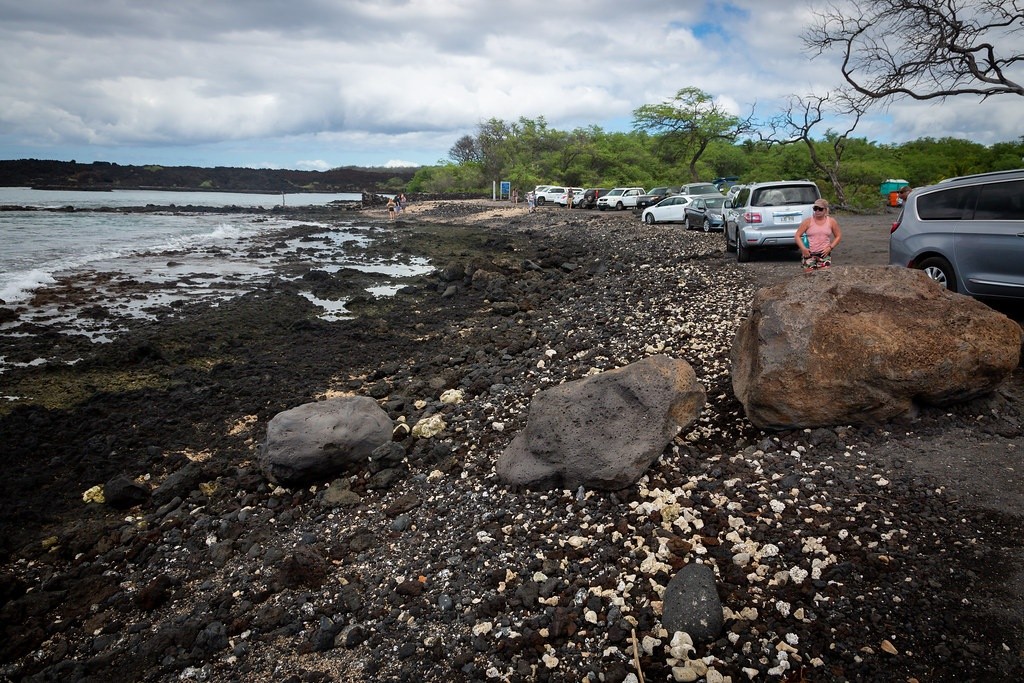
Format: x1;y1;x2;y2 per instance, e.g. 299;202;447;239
897;191;905;194
813;207;826;212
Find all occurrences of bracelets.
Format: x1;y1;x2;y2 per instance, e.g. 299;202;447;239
829;245;833;250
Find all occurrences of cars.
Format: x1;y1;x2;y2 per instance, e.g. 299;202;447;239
641;195;733;232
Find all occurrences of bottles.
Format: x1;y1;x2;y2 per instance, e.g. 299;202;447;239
803;235;809;247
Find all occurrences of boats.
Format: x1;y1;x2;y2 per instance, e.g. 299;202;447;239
32;170;113;192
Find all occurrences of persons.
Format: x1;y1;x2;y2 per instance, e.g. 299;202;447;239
899;185;912;206
386;192;408;220
794;198;843;270
513;185;574;214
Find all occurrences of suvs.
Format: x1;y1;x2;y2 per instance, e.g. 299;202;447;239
526;175;739;210
888;168;1024;306
723;179;821;261
